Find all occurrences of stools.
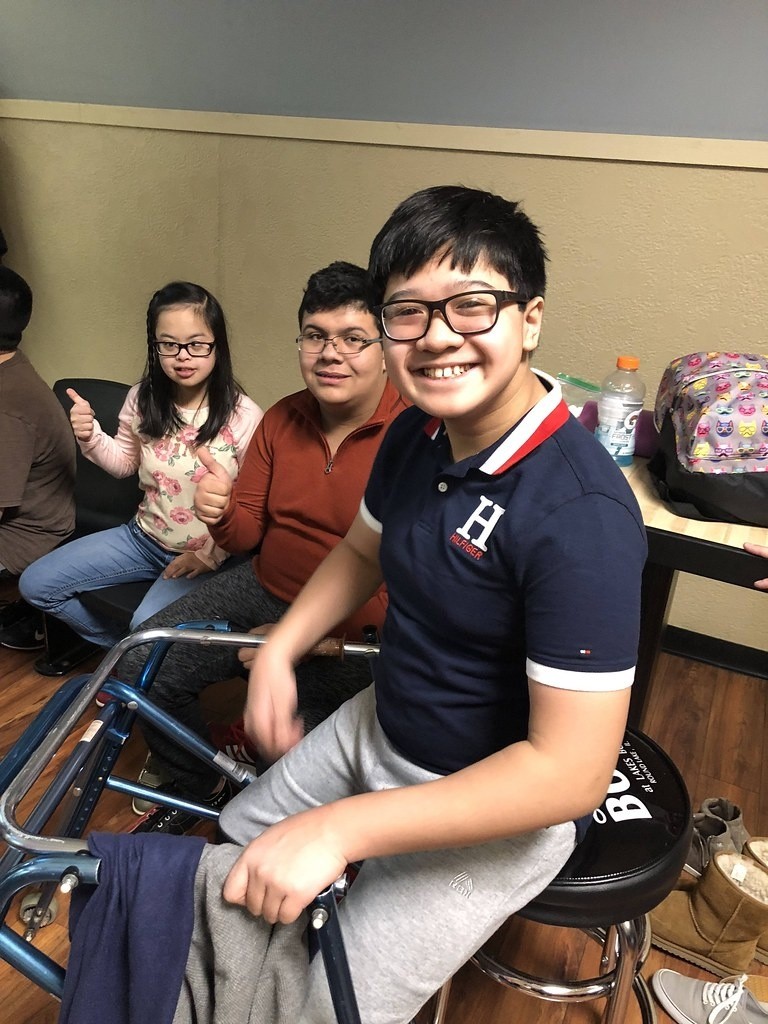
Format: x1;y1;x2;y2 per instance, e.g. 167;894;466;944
433;725;695;1024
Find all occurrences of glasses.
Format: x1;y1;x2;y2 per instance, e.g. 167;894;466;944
294;332;382;354
152;340;217;358
373;289;530;342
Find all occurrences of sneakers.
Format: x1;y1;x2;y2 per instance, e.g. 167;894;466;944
215;719;257;772
1;610;45;650
651;968;768;1024
129;776;233;837
0;595;33;630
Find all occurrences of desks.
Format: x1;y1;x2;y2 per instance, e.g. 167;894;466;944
619;454;768;731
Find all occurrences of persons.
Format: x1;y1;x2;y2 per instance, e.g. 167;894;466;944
0;266;76;575
117;261;415;835
216;186;650;1024
19;281;265;706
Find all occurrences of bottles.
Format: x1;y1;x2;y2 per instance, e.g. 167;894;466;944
591;356;645;467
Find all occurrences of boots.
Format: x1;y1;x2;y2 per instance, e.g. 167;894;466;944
741;835;768;965
646;851;768;980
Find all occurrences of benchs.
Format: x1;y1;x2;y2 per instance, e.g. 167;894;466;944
33;378;157;679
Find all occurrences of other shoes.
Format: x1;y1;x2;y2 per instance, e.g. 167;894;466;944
684;813;738;879
132;746;171;816
698;797;751;853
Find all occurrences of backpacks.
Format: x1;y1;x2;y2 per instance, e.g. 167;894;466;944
647;350;767;528
